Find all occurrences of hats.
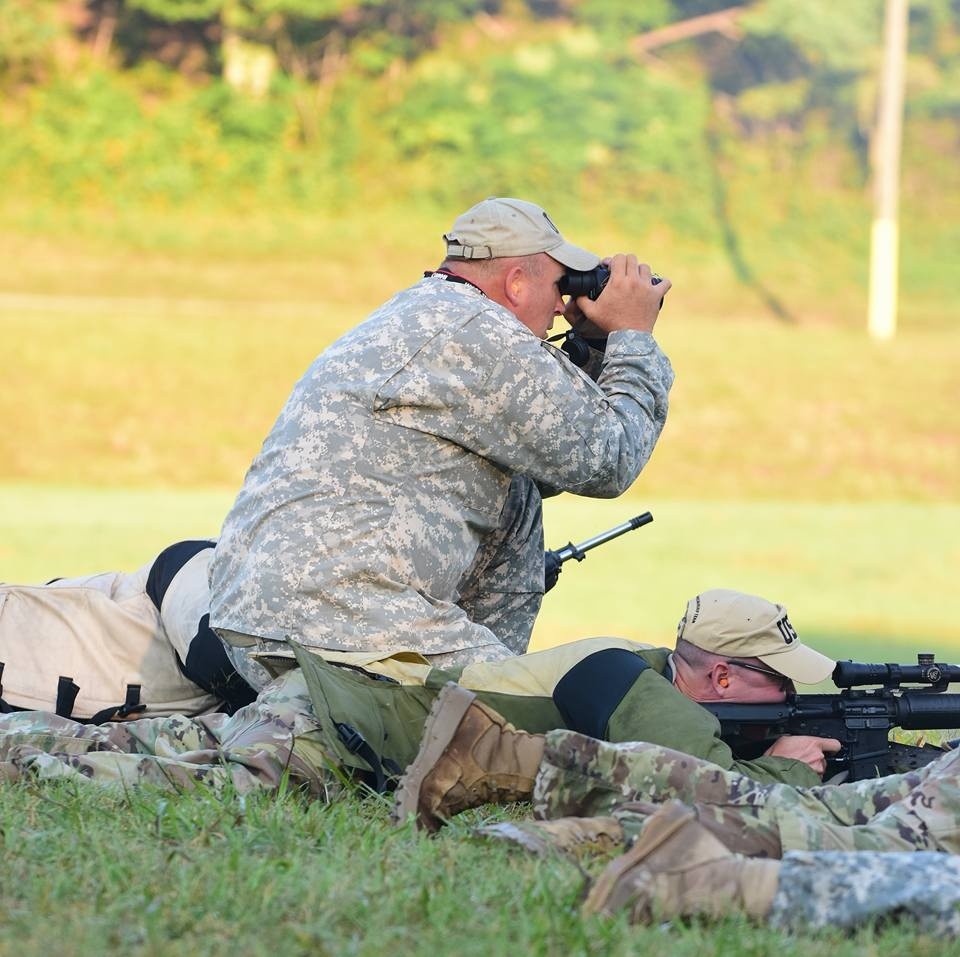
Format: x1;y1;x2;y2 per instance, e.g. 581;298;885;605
442;194;601;272
678;589;837;686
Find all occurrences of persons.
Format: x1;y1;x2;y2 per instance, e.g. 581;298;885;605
0;541;959;938
209;199;674;696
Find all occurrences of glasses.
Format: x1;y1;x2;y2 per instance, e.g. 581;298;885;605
728;660;791;694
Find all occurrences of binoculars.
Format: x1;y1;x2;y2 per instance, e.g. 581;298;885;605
558;265;664;309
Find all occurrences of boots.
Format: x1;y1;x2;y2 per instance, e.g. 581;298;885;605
393;681;548;840
472;814;627;902
579;799;783;957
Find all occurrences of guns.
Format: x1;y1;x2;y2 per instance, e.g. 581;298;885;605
693;652;960;783
541;511;654;595
